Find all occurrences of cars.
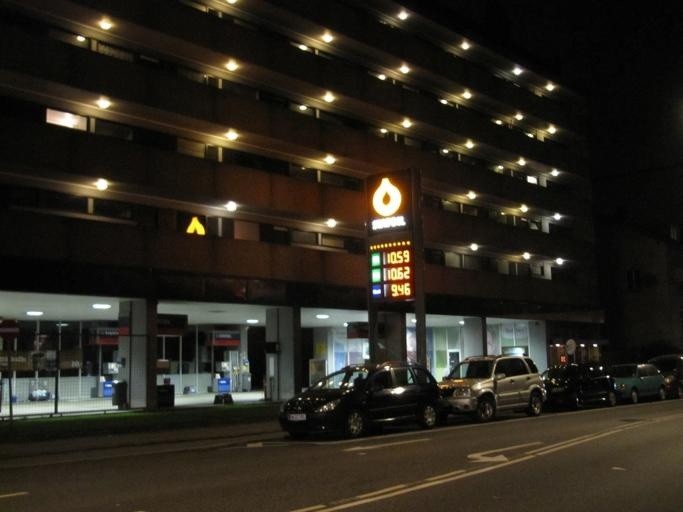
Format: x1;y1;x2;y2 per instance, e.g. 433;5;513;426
645;355;683;401
610;365;666;404
542;363;616;411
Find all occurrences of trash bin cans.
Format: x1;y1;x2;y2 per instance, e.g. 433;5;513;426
242;373;251;392
157;384;175;407
218;378;230;392
103;381;112;397
112;379;127;409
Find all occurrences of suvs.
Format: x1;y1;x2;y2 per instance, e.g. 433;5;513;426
277;362;446;442
438;353;548;424
